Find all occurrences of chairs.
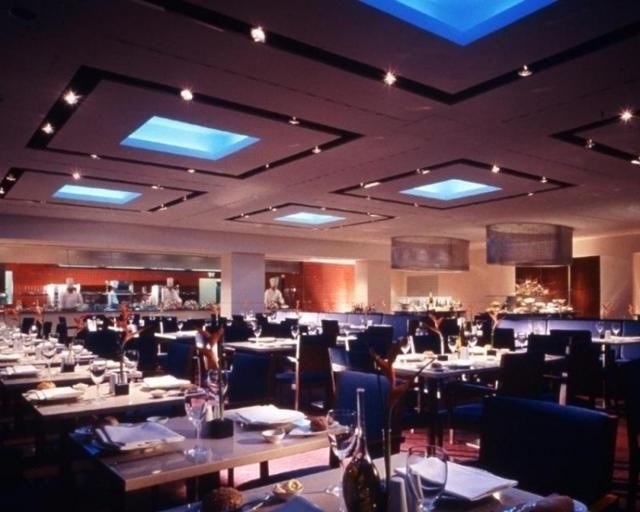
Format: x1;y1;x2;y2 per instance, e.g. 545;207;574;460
1;314;639;447
463;392;622;505
332;370;395;456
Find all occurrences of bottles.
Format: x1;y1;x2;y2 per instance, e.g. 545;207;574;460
344;388;384;511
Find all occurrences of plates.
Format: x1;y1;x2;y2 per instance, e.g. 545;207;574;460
395;455;515;502
235;405;305;428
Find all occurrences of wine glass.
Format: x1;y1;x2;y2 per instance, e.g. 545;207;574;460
447;335;459;365
177;320;184;337
252;324;263;349
89;360;105;403
207;369;228;424
594;320;622;340
405;445;447;511
182;388;211;457
398;336;411;367
325;410;365;495
467;332;478;359
123;350;140;395
517;332;528;355
344;324;351;339
3;324;85;376
290;324;298;347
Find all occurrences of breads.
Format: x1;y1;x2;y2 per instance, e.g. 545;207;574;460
536;496;574;511
310;417;328;431
37;382;55;390
180;384;198;391
201;487;244;512
96;416;118;428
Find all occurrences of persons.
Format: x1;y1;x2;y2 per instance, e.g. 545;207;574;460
60;278;83;309
163;277;183;307
264;276;286;311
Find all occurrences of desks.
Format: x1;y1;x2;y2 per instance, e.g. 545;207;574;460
161;451;591;512
68;403;351;503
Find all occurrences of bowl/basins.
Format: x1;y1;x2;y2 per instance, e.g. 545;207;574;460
261;429;286;445
271;480;302;502
145;415;169;429
72;382;89;393
150;389;166;399
